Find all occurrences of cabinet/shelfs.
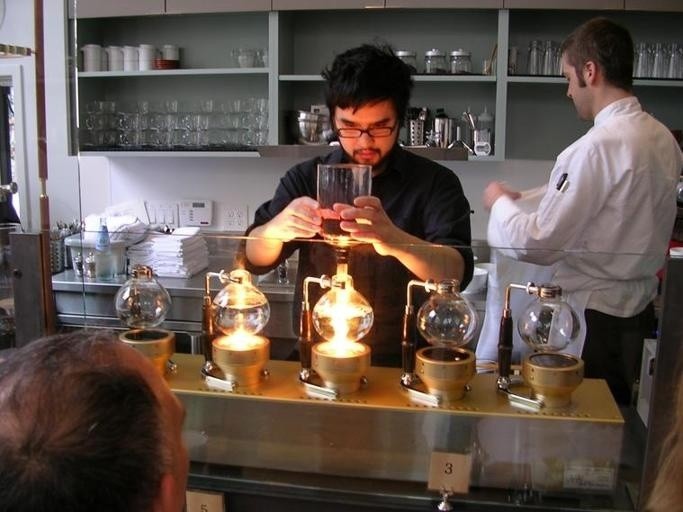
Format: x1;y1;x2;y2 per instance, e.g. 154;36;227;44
62;0;683;168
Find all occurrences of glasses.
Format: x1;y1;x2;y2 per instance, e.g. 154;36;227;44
333;111;398;138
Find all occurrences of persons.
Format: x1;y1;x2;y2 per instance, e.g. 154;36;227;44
231;39;478;375
480;14;681;408
0;329;190;511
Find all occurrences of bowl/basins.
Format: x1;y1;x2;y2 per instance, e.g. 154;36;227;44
296;110;331;144
449;263;489;295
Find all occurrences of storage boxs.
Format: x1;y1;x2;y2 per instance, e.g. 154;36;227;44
636;338;657;428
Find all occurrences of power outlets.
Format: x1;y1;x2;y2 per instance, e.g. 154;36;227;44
222;205;246;232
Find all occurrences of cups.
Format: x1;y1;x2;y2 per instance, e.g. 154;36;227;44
79;43;267;147
314;165;373;264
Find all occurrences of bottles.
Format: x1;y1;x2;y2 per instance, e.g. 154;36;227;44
75;217;118;281
390;48;496;156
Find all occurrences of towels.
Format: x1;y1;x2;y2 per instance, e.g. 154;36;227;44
83;211;210;279
